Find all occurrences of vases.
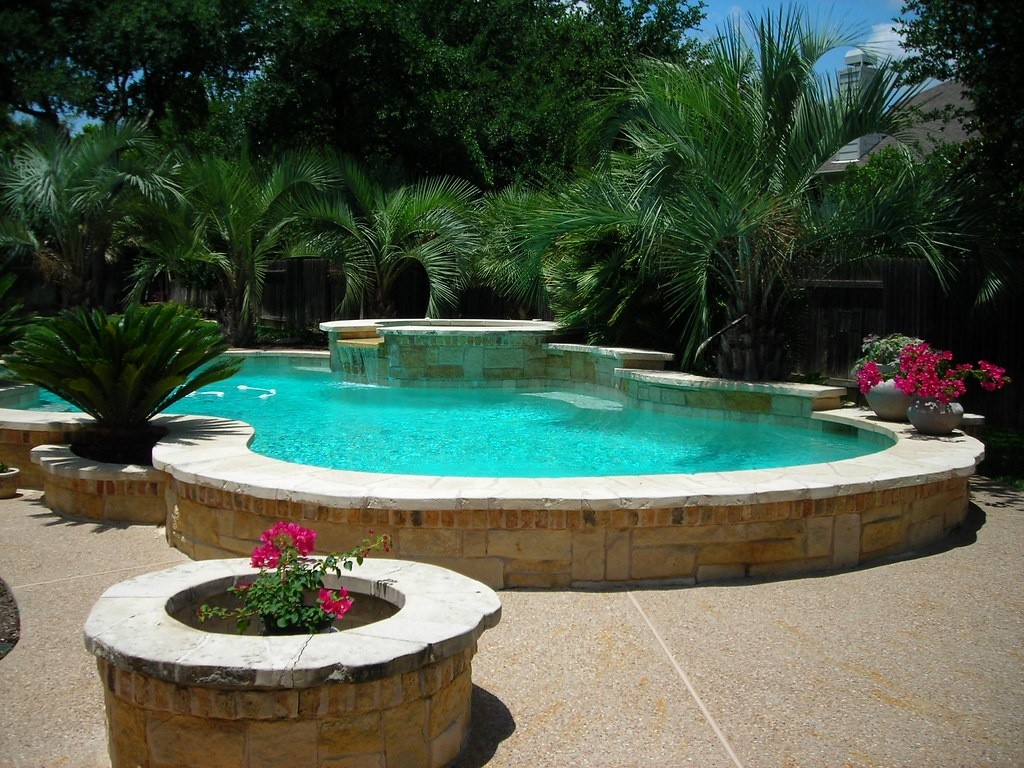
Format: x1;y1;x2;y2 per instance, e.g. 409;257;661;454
906;399;964;435
258;626;340;636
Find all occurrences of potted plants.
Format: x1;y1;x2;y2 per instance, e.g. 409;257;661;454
850;334;929;420
0;459;21;499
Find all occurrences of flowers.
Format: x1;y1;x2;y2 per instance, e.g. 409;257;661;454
197;518;395;633
855;344;1013;401
